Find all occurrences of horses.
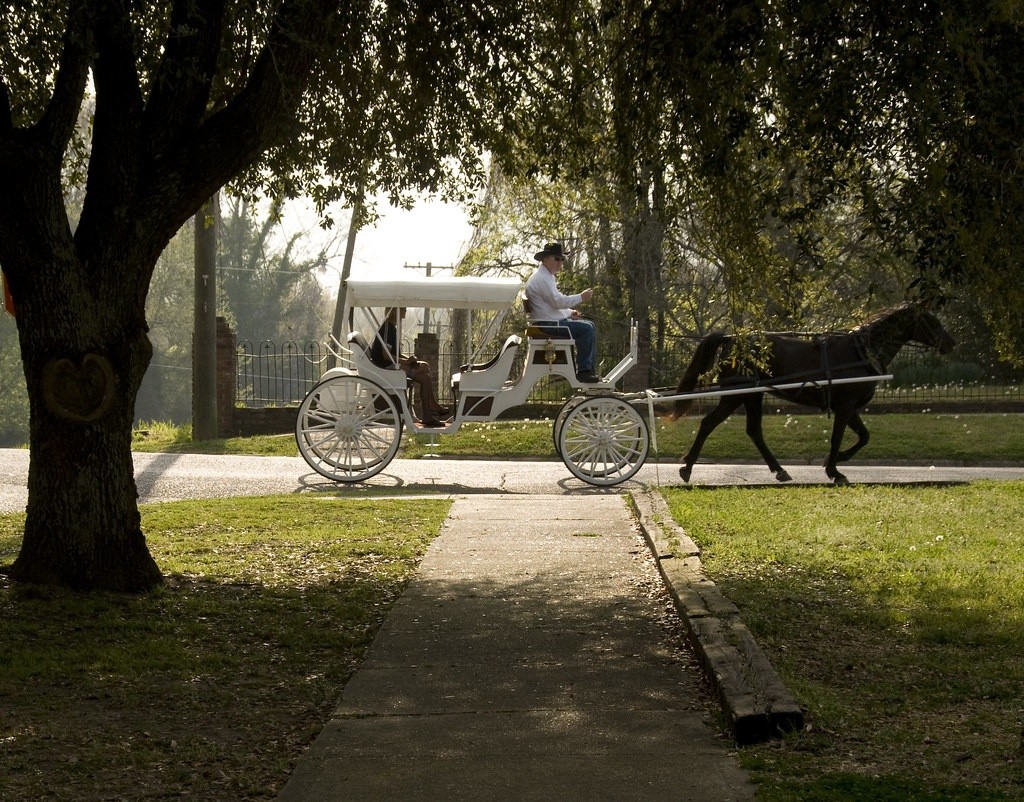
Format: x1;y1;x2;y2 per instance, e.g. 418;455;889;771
660;295;957;487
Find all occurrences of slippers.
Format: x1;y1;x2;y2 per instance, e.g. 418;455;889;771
421;419;445;427
432;408;449;416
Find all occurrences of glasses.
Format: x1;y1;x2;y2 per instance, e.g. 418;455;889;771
548;256;565;261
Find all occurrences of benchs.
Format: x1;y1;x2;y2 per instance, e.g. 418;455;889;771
521;291;571;340
451;335;522;392
347;331;413;390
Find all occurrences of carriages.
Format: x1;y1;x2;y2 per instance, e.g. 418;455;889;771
294;274;959;487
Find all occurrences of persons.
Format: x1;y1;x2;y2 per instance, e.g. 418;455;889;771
525;244;598;383
370;307;451;428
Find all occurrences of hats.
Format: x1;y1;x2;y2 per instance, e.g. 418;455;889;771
534;243;570;261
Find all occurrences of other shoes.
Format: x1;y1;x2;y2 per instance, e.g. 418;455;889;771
579;370;600;382
